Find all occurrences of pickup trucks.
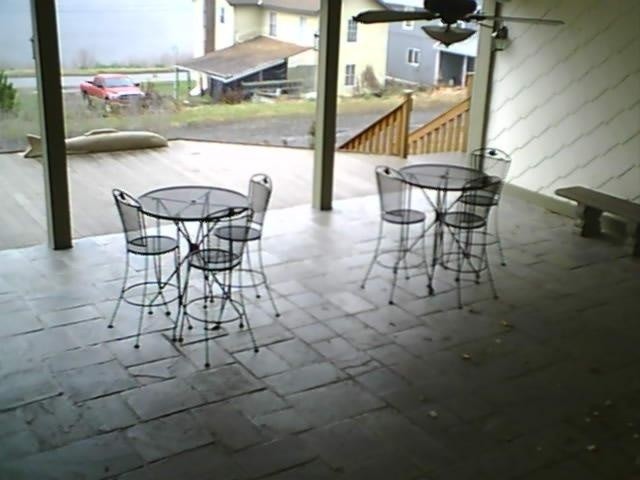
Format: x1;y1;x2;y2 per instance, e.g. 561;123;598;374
79;72;148;115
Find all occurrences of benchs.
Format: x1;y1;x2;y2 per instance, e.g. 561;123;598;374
554;185;639;256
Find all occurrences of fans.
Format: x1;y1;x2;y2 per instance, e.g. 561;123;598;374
351;0;566;48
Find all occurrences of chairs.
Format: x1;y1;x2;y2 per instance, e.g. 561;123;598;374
107;173;281;367
359;149;513;310
